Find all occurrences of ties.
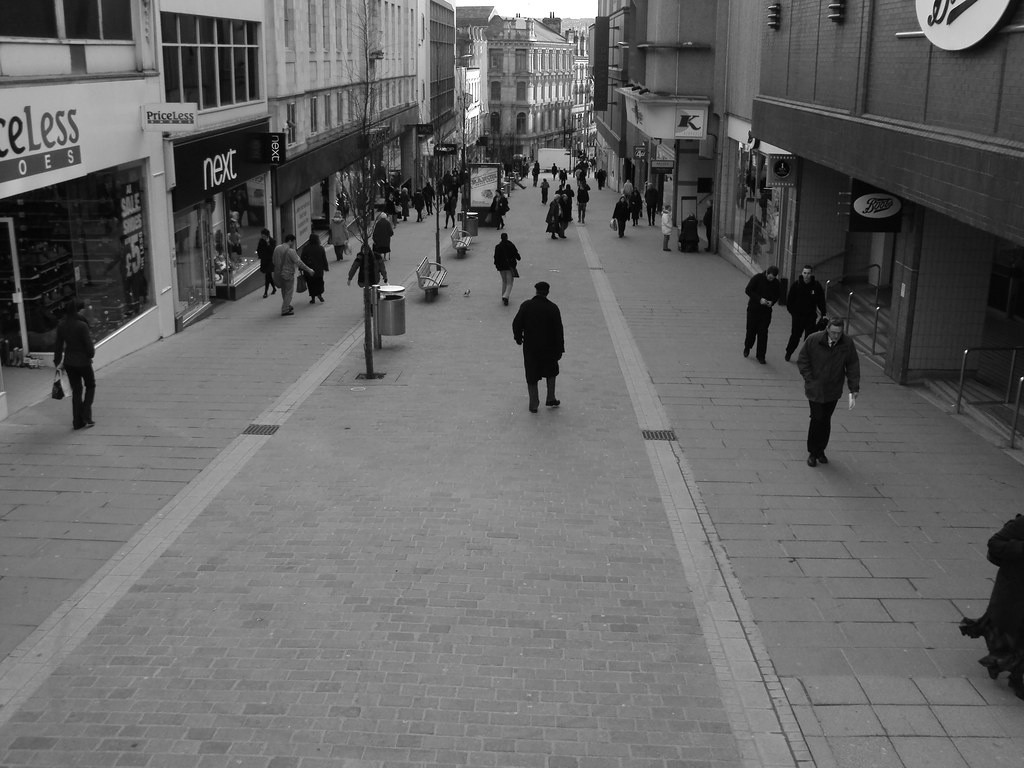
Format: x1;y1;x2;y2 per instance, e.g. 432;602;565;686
831;341;835;347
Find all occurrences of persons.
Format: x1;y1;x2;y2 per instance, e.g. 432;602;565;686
272;234;315;316
51;296;96;430
494;233;521;305
797;319;861;467
743;265;782;365
347;243;389;316
678;213;700;253
298;234;330;304
532;154;643;240
785;265;829;365
703;199;713;249
372;212;394;259
329;210;349;261
645;182;659;226
387;145;520;228
256;228;277;298
512;280;565;413
737;166;756;204
765;206;779;254
959;513;1024;703
511;173;528;189
660;205;672;251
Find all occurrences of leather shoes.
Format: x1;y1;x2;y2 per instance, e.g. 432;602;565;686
808;452;817;467
818;450;828;463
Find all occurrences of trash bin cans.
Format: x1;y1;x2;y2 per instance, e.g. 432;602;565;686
464;212;479;237
509;177;515;190
503;182;511;193
514;172;519;182
378;285;406;336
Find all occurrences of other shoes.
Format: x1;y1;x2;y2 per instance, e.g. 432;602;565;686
663;248;671;251
1008;673;1024;700
744;345;750;357
988;666;998;678
498;228;499;230
501;223;505;229
756;354;767;365
502;298;509;306
546;398;560;406
552;235;558;239
785;348;791;362
263;289;324;316
74;416;95;430
529;407;538;414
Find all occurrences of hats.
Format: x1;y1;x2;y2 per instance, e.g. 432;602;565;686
380;211;387;218
534;281;550;291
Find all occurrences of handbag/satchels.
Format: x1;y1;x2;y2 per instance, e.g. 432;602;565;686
817;314;829;331
343;241;351;255
610;218;617;231
273;267;282;288
510;267;519;279
52;369;72;400
297;270;306;293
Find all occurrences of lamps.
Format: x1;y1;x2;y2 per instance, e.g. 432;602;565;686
767;3;781;31
624;84;650;95
827;0;845;23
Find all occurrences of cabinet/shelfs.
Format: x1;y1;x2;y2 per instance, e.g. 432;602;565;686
0;196;136;337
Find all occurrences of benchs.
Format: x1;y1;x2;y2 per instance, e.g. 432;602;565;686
450;226;473;260
416;256;448;303
